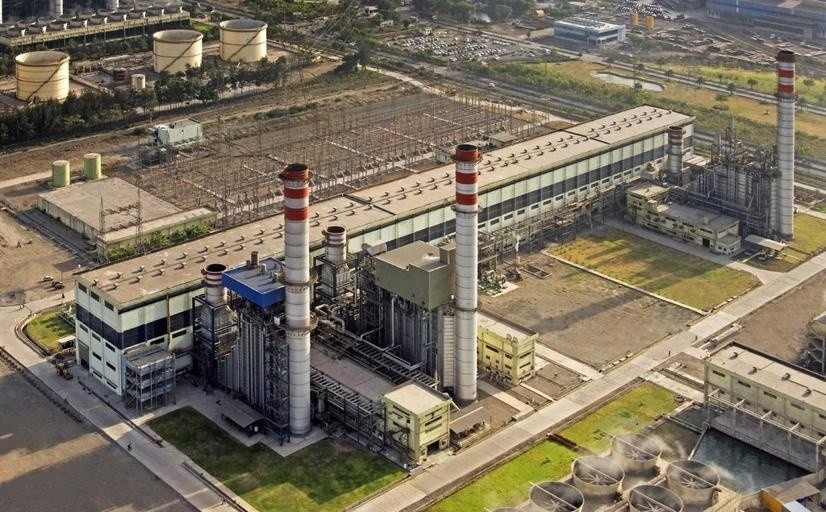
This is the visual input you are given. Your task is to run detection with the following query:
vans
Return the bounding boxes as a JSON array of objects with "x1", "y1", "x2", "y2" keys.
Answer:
[
  {"x1": 52, "y1": 281, "x2": 63, "y2": 287},
  {"x1": 54, "y1": 283, "x2": 65, "y2": 290},
  {"x1": 43, "y1": 276, "x2": 54, "y2": 282}
]
